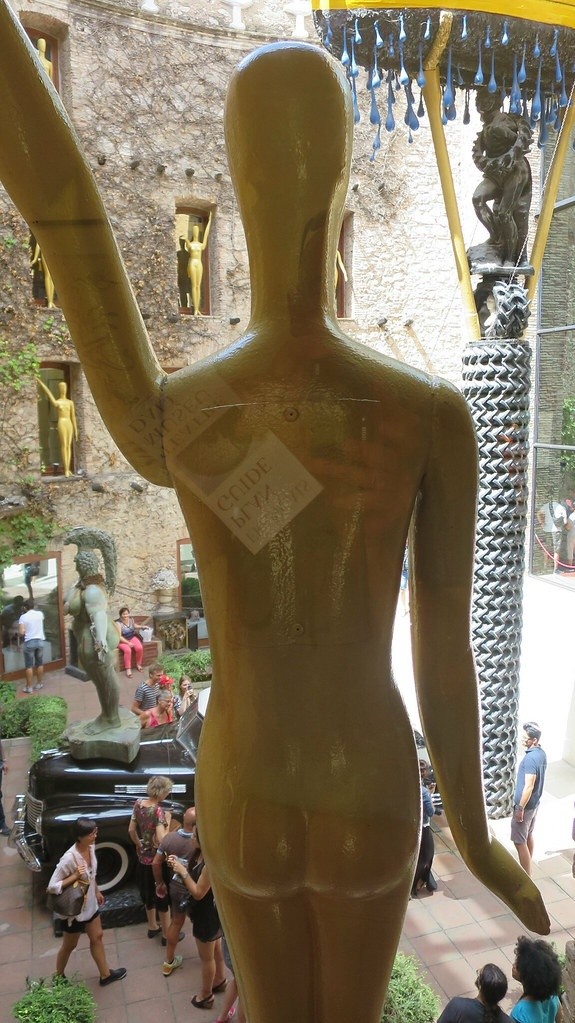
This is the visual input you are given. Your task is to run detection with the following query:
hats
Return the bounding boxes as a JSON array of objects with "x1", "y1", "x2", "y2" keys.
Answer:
[{"x1": 158, "y1": 675, "x2": 174, "y2": 685}]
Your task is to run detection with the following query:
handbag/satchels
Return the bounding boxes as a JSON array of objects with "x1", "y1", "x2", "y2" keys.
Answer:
[
  {"x1": 45, "y1": 851, "x2": 92, "y2": 917},
  {"x1": 553, "y1": 516, "x2": 564, "y2": 528},
  {"x1": 133, "y1": 631, "x2": 143, "y2": 642}
]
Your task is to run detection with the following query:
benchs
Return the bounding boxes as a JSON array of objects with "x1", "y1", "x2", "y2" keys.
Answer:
[{"x1": 112, "y1": 641, "x2": 163, "y2": 673}]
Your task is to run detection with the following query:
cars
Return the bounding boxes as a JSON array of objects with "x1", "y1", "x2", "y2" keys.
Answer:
[{"x1": 4, "y1": 685, "x2": 445, "y2": 898}]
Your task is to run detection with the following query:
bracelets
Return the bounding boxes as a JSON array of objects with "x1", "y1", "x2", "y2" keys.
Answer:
[
  {"x1": 3, "y1": 759, "x2": 5, "y2": 762},
  {"x1": 155, "y1": 882, "x2": 163, "y2": 887},
  {"x1": 181, "y1": 872, "x2": 189, "y2": 879}
]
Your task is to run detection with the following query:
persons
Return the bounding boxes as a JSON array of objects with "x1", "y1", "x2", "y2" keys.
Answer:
[
  {"x1": 411, "y1": 759, "x2": 438, "y2": 897},
  {"x1": 1, "y1": 596, "x2": 24, "y2": 649},
  {"x1": 538, "y1": 489, "x2": 575, "y2": 574},
  {"x1": 47, "y1": 816, "x2": 126, "y2": 989},
  {"x1": 400, "y1": 539, "x2": 409, "y2": 589},
  {"x1": 335, "y1": 251, "x2": 347, "y2": 288},
  {"x1": 19, "y1": 563, "x2": 33, "y2": 600},
  {"x1": 129, "y1": 775, "x2": 238, "y2": 1023},
  {"x1": 62, "y1": 526, "x2": 121, "y2": 735},
  {"x1": 131, "y1": 664, "x2": 195, "y2": 729},
  {"x1": 16, "y1": 599, "x2": 44, "y2": 693},
  {"x1": 436, "y1": 935, "x2": 562, "y2": 1023},
  {"x1": 510, "y1": 721, "x2": 547, "y2": 876},
  {"x1": 37, "y1": 377, "x2": 78, "y2": 478},
  {"x1": 0, "y1": 0, "x2": 547, "y2": 1023},
  {"x1": 180, "y1": 210, "x2": 211, "y2": 315},
  {"x1": 471, "y1": 90, "x2": 535, "y2": 258},
  {"x1": 115, "y1": 608, "x2": 149, "y2": 677},
  {"x1": 0, "y1": 703, "x2": 12, "y2": 835},
  {"x1": 31, "y1": 242, "x2": 56, "y2": 309}
]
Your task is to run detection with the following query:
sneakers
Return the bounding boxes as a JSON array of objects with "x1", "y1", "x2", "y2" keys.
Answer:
[
  {"x1": 100, "y1": 967, "x2": 127, "y2": 986},
  {"x1": 161, "y1": 954, "x2": 183, "y2": 976},
  {"x1": 55, "y1": 974, "x2": 68, "y2": 983},
  {"x1": 214, "y1": 1007, "x2": 235, "y2": 1023}
]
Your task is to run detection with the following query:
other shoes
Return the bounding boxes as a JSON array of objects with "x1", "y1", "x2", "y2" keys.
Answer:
[
  {"x1": 544, "y1": 565, "x2": 548, "y2": 569},
  {"x1": 126, "y1": 670, "x2": 133, "y2": 678},
  {"x1": 33, "y1": 683, "x2": 44, "y2": 690},
  {"x1": 136, "y1": 665, "x2": 144, "y2": 672},
  {"x1": 553, "y1": 569, "x2": 564, "y2": 574},
  {"x1": 148, "y1": 924, "x2": 162, "y2": 938},
  {"x1": 23, "y1": 687, "x2": 33, "y2": 692},
  {"x1": 0, "y1": 825, "x2": 13, "y2": 835},
  {"x1": 162, "y1": 932, "x2": 185, "y2": 946}
]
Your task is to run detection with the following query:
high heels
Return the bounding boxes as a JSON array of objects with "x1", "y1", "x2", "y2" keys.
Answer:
[
  {"x1": 191, "y1": 993, "x2": 215, "y2": 1009},
  {"x1": 212, "y1": 978, "x2": 228, "y2": 992}
]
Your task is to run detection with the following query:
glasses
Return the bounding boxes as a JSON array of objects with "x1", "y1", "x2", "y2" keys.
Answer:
[{"x1": 522, "y1": 735, "x2": 536, "y2": 740}]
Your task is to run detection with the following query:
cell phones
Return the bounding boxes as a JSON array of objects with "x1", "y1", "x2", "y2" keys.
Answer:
[
  {"x1": 188, "y1": 685, "x2": 192, "y2": 690},
  {"x1": 179, "y1": 858, "x2": 187, "y2": 867}
]
